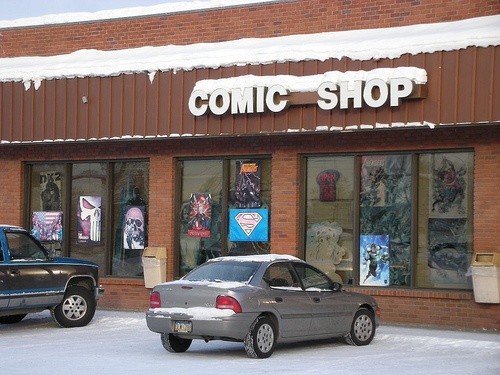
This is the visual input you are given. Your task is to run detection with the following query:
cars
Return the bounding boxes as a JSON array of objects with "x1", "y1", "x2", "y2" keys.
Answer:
[
  {"x1": 147, "y1": 253, "x2": 382, "y2": 355},
  {"x1": 1, "y1": 224, "x2": 104, "y2": 329}
]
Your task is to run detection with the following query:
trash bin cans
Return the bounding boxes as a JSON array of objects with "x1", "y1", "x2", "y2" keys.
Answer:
[
  {"x1": 470, "y1": 250, "x2": 500, "y2": 303},
  {"x1": 141, "y1": 246, "x2": 167, "y2": 289}
]
